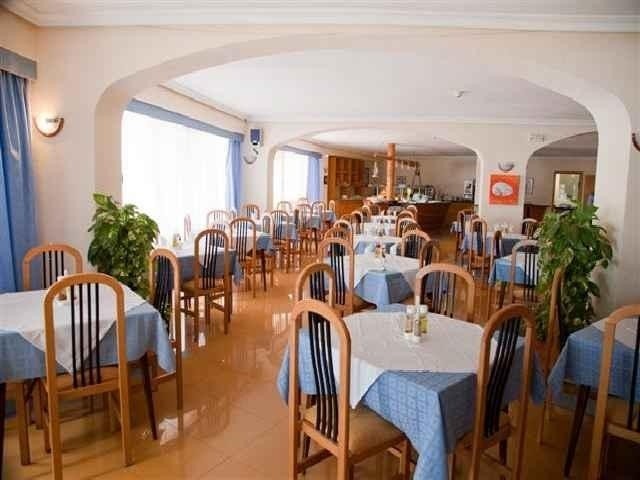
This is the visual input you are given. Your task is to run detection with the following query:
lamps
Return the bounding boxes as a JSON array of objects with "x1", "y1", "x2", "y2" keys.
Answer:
[
  {"x1": 32, "y1": 111, "x2": 65, "y2": 136},
  {"x1": 244, "y1": 155, "x2": 258, "y2": 165},
  {"x1": 498, "y1": 160, "x2": 515, "y2": 173}
]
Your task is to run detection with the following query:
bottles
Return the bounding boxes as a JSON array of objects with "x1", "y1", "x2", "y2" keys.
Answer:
[
  {"x1": 170, "y1": 231, "x2": 181, "y2": 248},
  {"x1": 57, "y1": 268, "x2": 78, "y2": 303},
  {"x1": 374, "y1": 245, "x2": 387, "y2": 260},
  {"x1": 404, "y1": 304, "x2": 430, "y2": 343}
]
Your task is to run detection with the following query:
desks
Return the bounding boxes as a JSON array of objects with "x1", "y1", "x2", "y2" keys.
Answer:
[
  {"x1": 296, "y1": 306, "x2": 524, "y2": 479},
  {"x1": 1, "y1": 281, "x2": 160, "y2": 444}
]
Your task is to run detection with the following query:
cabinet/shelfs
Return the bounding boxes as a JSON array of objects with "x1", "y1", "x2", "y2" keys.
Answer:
[{"x1": 328, "y1": 152, "x2": 368, "y2": 205}]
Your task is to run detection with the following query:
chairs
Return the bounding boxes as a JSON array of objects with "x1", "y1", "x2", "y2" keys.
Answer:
[
  {"x1": 293, "y1": 264, "x2": 338, "y2": 325},
  {"x1": 444, "y1": 302, "x2": 531, "y2": 480},
  {"x1": 4, "y1": 378, "x2": 32, "y2": 466},
  {"x1": 37, "y1": 272, "x2": 133, "y2": 479},
  {"x1": 19, "y1": 243, "x2": 83, "y2": 431},
  {"x1": 534, "y1": 264, "x2": 639, "y2": 480},
  {"x1": 98, "y1": 246, "x2": 181, "y2": 431},
  {"x1": 141, "y1": 196, "x2": 548, "y2": 342},
  {"x1": 288, "y1": 300, "x2": 409, "y2": 479}
]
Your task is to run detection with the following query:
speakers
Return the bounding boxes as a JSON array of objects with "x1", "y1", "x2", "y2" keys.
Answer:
[{"x1": 248, "y1": 128, "x2": 262, "y2": 148}]
